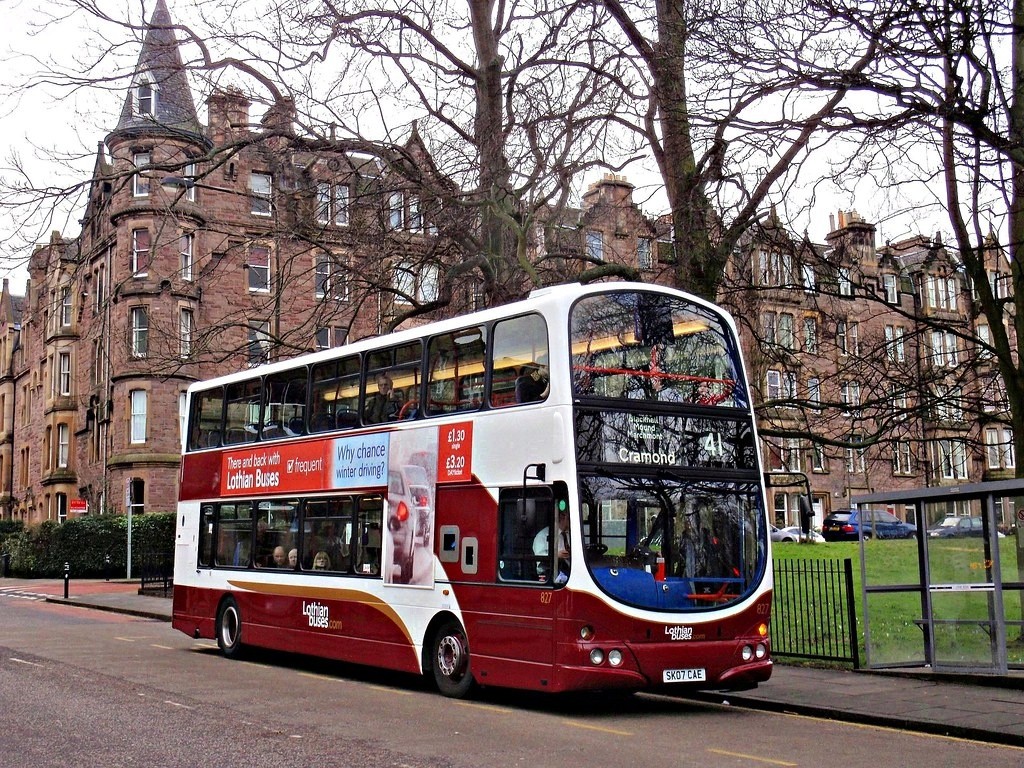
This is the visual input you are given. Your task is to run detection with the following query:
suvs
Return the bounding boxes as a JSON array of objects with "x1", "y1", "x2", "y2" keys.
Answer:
[{"x1": 821, "y1": 507, "x2": 917, "y2": 542}]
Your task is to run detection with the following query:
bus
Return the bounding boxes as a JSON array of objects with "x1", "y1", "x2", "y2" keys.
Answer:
[{"x1": 171, "y1": 281, "x2": 816, "y2": 706}]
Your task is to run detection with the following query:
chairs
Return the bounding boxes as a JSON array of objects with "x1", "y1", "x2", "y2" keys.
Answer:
[{"x1": 190, "y1": 375, "x2": 742, "y2": 608}]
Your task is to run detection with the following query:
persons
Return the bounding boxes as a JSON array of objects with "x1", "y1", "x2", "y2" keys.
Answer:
[
  {"x1": 324, "y1": 525, "x2": 344, "y2": 547},
  {"x1": 312, "y1": 553, "x2": 331, "y2": 571},
  {"x1": 515, "y1": 355, "x2": 550, "y2": 404},
  {"x1": 687, "y1": 381, "x2": 732, "y2": 405},
  {"x1": 272, "y1": 545, "x2": 287, "y2": 569},
  {"x1": 532, "y1": 504, "x2": 570, "y2": 582},
  {"x1": 287, "y1": 549, "x2": 298, "y2": 569},
  {"x1": 367, "y1": 376, "x2": 404, "y2": 423}
]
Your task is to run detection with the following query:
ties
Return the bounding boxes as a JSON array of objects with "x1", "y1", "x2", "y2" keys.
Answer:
[{"x1": 561, "y1": 532, "x2": 571, "y2": 553}]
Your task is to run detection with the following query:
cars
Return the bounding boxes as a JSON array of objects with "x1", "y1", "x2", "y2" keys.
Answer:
[
  {"x1": 770, "y1": 524, "x2": 797, "y2": 542},
  {"x1": 781, "y1": 526, "x2": 826, "y2": 542},
  {"x1": 926, "y1": 515, "x2": 983, "y2": 539}
]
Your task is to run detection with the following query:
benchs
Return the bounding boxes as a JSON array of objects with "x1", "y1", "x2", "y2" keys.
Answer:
[{"x1": 912, "y1": 619, "x2": 1024, "y2": 675}]
